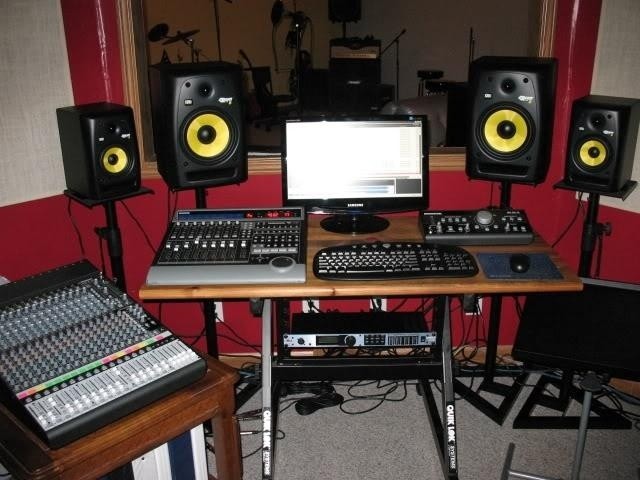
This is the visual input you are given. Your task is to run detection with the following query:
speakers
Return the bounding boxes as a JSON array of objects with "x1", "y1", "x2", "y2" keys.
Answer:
[
  {"x1": 148, "y1": 62, "x2": 248, "y2": 189},
  {"x1": 564, "y1": 95, "x2": 640, "y2": 191},
  {"x1": 330, "y1": 40, "x2": 381, "y2": 113},
  {"x1": 56, "y1": 102, "x2": 142, "y2": 200},
  {"x1": 461, "y1": 56, "x2": 559, "y2": 186}
]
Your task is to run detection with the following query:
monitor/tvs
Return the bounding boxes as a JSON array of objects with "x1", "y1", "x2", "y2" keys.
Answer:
[{"x1": 280, "y1": 114, "x2": 429, "y2": 235}]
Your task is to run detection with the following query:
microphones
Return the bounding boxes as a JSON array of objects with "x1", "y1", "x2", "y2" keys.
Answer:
[{"x1": 396, "y1": 26, "x2": 406, "y2": 39}]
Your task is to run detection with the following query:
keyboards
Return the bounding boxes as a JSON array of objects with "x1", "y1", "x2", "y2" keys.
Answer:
[{"x1": 313, "y1": 242, "x2": 479, "y2": 278}]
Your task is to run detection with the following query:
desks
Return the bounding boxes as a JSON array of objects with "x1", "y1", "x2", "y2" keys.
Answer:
[
  {"x1": 138, "y1": 209, "x2": 586, "y2": 480},
  {"x1": 2, "y1": 347, "x2": 245, "y2": 480}
]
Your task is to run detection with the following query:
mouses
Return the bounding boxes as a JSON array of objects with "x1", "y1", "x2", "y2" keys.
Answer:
[{"x1": 510, "y1": 253, "x2": 531, "y2": 273}]
